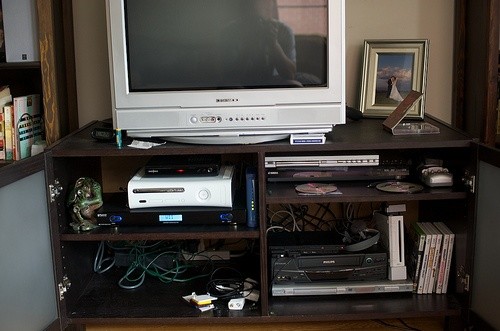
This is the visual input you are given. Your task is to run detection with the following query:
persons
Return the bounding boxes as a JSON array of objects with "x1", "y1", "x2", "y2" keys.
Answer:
[
  {"x1": 386, "y1": 76, "x2": 405, "y2": 101},
  {"x1": 228, "y1": 0, "x2": 302, "y2": 88}
]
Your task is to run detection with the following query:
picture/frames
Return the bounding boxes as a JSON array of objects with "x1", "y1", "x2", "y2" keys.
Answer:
[{"x1": 359, "y1": 39, "x2": 430, "y2": 120}]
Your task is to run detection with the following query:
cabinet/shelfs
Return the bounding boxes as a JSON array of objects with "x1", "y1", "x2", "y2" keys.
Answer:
[{"x1": 43, "y1": 112, "x2": 482, "y2": 331}]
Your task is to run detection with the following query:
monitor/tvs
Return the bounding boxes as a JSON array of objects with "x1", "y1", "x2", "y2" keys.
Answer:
[{"x1": 104, "y1": 0, "x2": 346, "y2": 145}]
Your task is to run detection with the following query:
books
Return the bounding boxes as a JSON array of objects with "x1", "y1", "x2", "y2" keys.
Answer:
[
  {"x1": 0, "y1": 86, "x2": 43, "y2": 161},
  {"x1": 411, "y1": 219, "x2": 455, "y2": 295}
]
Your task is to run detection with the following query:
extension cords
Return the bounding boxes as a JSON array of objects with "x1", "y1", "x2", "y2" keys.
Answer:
[{"x1": 179, "y1": 249, "x2": 231, "y2": 261}]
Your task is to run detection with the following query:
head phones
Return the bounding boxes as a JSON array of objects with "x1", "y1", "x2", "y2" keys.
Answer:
[{"x1": 333, "y1": 220, "x2": 381, "y2": 252}]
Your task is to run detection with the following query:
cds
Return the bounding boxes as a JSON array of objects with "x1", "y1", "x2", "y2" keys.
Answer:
[
  {"x1": 296, "y1": 183, "x2": 338, "y2": 193},
  {"x1": 376, "y1": 181, "x2": 422, "y2": 193}
]
